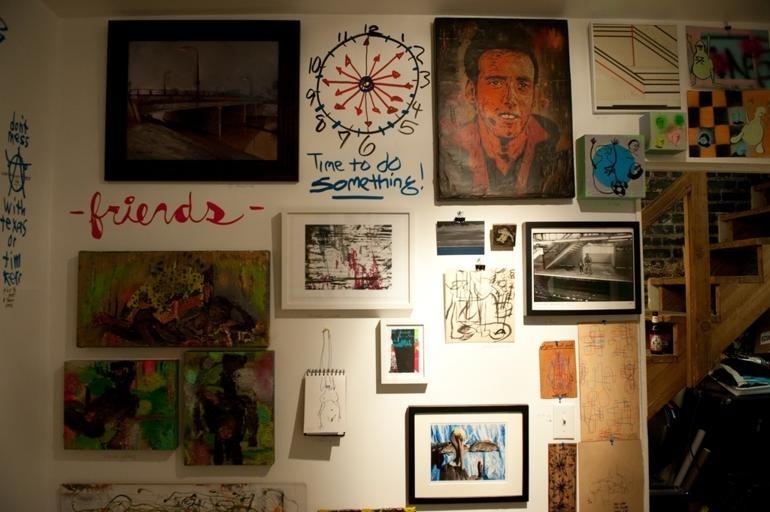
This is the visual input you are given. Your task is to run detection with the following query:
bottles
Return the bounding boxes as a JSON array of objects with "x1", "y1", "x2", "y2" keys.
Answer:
[{"x1": 648, "y1": 310, "x2": 664, "y2": 355}]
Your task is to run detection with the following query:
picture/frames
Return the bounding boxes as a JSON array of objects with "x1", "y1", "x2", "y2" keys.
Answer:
[
  {"x1": 103, "y1": 16, "x2": 302, "y2": 188},
  {"x1": 406, "y1": 404, "x2": 530, "y2": 506},
  {"x1": 378, "y1": 319, "x2": 429, "y2": 387},
  {"x1": 521, "y1": 221, "x2": 644, "y2": 318},
  {"x1": 278, "y1": 205, "x2": 416, "y2": 309}
]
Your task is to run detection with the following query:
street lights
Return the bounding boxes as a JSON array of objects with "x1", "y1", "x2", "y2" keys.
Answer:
[
  {"x1": 163, "y1": 70, "x2": 171, "y2": 95},
  {"x1": 243, "y1": 77, "x2": 253, "y2": 96},
  {"x1": 176, "y1": 45, "x2": 199, "y2": 96}
]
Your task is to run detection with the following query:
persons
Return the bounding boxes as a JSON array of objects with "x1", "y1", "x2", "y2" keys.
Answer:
[
  {"x1": 578, "y1": 258, "x2": 584, "y2": 273},
  {"x1": 584, "y1": 252, "x2": 593, "y2": 276},
  {"x1": 448, "y1": 22, "x2": 562, "y2": 197},
  {"x1": 317, "y1": 377, "x2": 341, "y2": 431}
]
